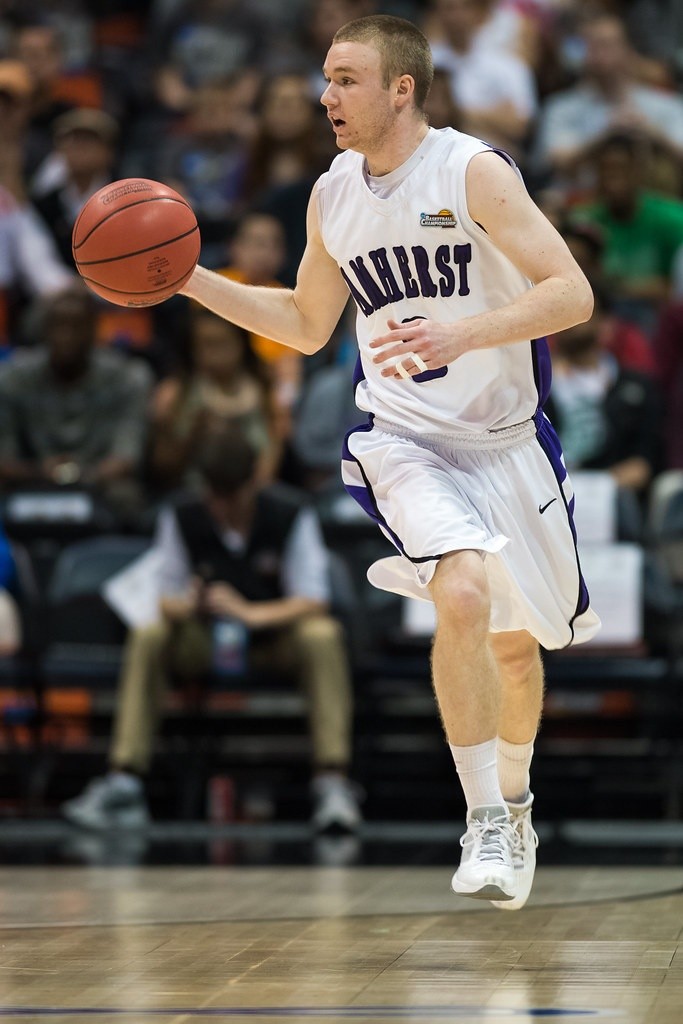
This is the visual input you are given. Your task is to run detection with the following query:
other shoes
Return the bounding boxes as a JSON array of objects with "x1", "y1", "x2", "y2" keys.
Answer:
[
  {"x1": 62, "y1": 767, "x2": 149, "y2": 828},
  {"x1": 312, "y1": 775, "x2": 362, "y2": 836}
]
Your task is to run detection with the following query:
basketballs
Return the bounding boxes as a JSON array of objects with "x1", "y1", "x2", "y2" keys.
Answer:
[{"x1": 72, "y1": 179, "x2": 202, "y2": 309}]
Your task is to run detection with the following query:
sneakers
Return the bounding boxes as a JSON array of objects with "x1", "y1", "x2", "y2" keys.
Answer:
[
  {"x1": 452, "y1": 804, "x2": 520, "y2": 900},
  {"x1": 491, "y1": 791, "x2": 539, "y2": 911}
]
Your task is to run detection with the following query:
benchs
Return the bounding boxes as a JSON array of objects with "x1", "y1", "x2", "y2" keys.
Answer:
[{"x1": 3, "y1": 686, "x2": 630, "y2": 866}]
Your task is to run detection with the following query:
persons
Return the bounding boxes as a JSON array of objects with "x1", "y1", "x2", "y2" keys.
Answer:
[
  {"x1": 174, "y1": 15, "x2": 596, "y2": 911},
  {"x1": 0, "y1": 0, "x2": 682, "y2": 837}
]
